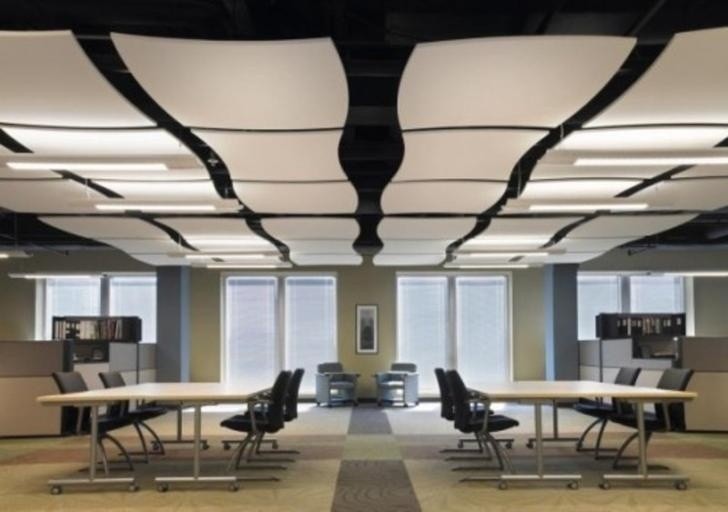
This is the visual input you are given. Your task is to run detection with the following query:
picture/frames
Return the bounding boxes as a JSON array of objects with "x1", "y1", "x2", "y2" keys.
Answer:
[{"x1": 353, "y1": 303, "x2": 379, "y2": 356}]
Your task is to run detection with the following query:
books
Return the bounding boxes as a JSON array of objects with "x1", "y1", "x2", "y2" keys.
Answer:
[
  {"x1": 615, "y1": 315, "x2": 683, "y2": 338},
  {"x1": 54, "y1": 319, "x2": 135, "y2": 341}
]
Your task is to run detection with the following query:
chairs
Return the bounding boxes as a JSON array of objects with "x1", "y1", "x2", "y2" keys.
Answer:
[
  {"x1": 37, "y1": 359, "x2": 307, "y2": 497},
  {"x1": 430, "y1": 364, "x2": 698, "y2": 494},
  {"x1": 373, "y1": 361, "x2": 422, "y2": 409},
  {"x1": 314, "y1": 360, "x2": 362, "y2": 409}
]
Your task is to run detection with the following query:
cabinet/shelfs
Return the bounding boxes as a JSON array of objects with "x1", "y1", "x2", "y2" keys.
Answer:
[
  {"x1": 594, "y1": 310, "x2": 686, "y2": 357},
  {"x1": 50, "y1": 315, "x2": 142, "y2": 363}
]
